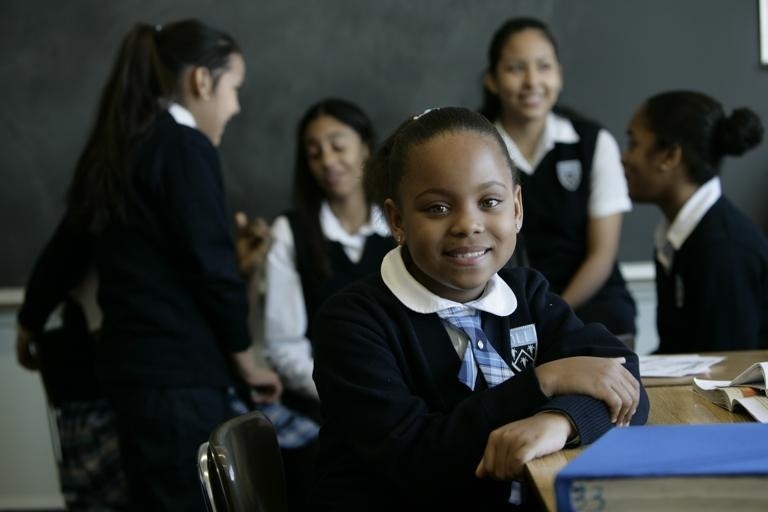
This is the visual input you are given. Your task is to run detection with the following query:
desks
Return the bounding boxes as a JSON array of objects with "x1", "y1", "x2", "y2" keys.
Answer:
[{"x1": 523, "y1": 349, "x2": 767, "y2": 512}]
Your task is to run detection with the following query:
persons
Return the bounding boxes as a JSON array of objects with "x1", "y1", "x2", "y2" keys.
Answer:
[
  {"x1": 473, "y1": 14, "x2": 638, "y2": 352},
  {"x1": 308, "y1": 104, "x2": 652, "y2": 511},
  {"x1": 261, "y1": 95, "x2": 404, "y2": 419},
  {"x1": 60, "y1": 210, "x2": 280, "y2": 344},
  {"x1": 620, "y1": 86, "x2": 768, "y2": 353},
  {"x1": 12, "y1": 15, "x2": 286, "y2": 511}
]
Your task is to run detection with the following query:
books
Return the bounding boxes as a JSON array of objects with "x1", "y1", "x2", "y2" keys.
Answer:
[{"x1": 553, "y1": 350, "x2": 768, "y2": 512}]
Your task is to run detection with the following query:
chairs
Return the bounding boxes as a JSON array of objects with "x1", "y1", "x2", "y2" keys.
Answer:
[{"x1": 195, "y1": 411, "x2": 286, "y2": 512}]
